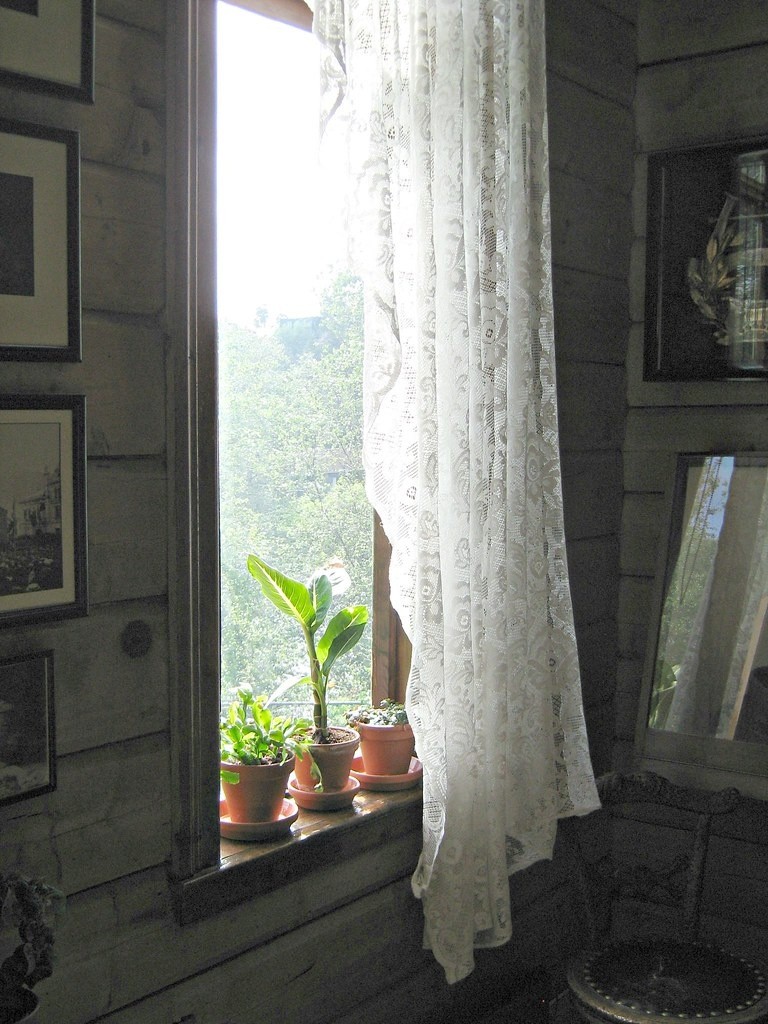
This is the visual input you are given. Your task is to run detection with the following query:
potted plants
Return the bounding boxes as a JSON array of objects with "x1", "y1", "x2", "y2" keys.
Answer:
[{"x1": 218, "y1": 551, "x2": 425, "y2": 843}]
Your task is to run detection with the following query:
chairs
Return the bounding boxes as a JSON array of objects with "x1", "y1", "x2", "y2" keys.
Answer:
[{"x1": 567, "y1": 766, "x2": 768, "y2": 1024}]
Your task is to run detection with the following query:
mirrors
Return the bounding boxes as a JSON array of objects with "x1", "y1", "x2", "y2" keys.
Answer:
[{"x1": 632, "y1": 450, "x2": 768, "y2": 800}]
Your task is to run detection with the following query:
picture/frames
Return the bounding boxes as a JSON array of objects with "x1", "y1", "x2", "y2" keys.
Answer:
[
  {"x1": 623, "y1": 123, "x2": 768, "y2": 407},
  {"x1": 0, "y1": 0, "x2": 96, "y2": 107},
  {"x1": 0, "y1": 647, "x2": 58, "y2": 808},
  {"x1": 0, "y1": 393, "x2": 90, "y2": 629},
  {"x1": 0, "y1": 118, "x2": 82, "y2": 363}
]
